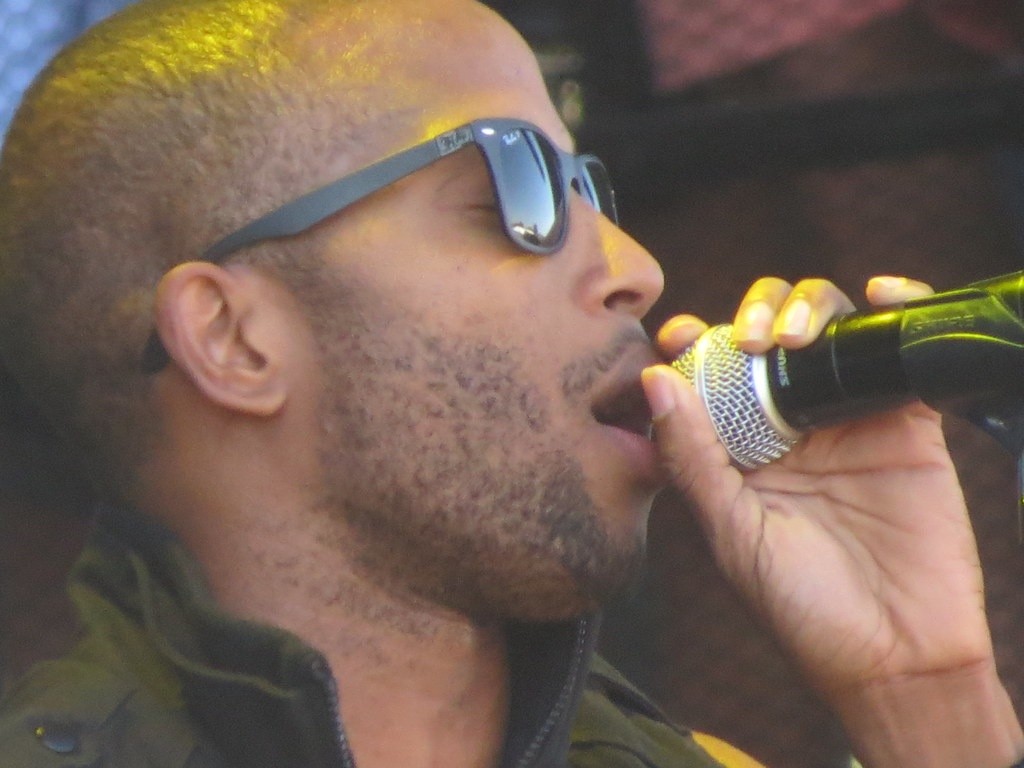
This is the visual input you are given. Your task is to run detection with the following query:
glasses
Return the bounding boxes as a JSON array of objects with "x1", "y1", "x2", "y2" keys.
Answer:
[{"x1": 133, "y1": 117, "x2": 620, "y2": 373}]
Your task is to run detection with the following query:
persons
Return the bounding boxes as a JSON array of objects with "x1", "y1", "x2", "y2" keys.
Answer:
[{"x1": 0, "y1": 0, "x2": 1024, "y2": 768}]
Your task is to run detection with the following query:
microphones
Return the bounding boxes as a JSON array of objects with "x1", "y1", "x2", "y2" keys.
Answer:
[{"x1": 647, "y1": 271, "x2": 1024, "y2": 475}]
